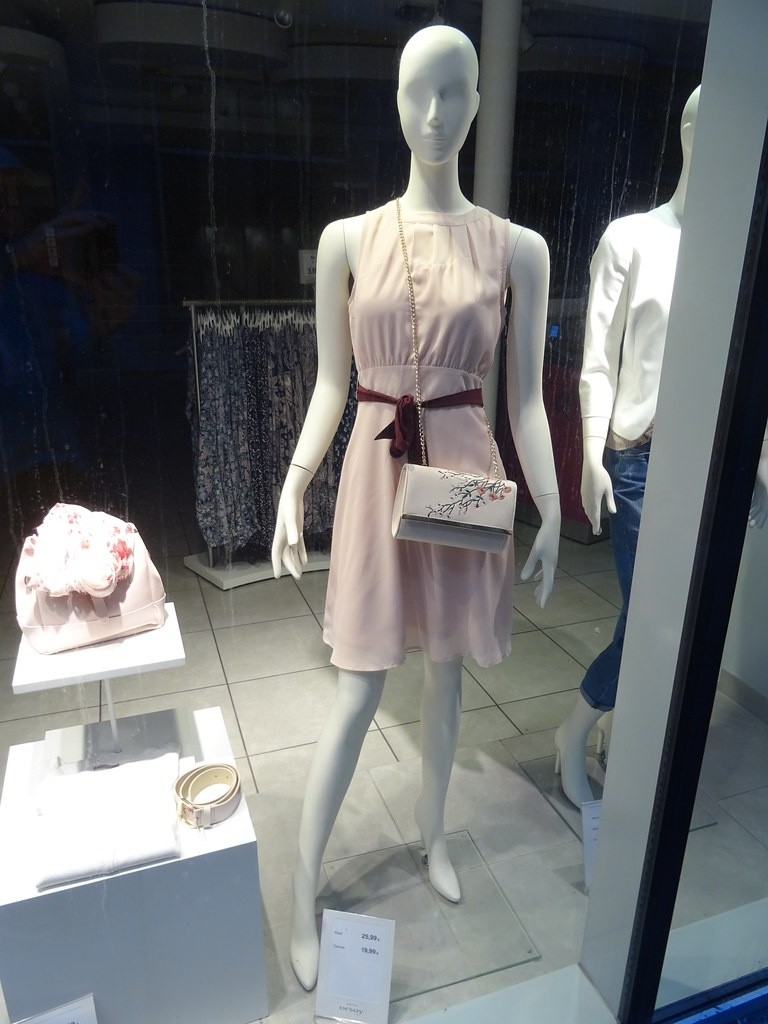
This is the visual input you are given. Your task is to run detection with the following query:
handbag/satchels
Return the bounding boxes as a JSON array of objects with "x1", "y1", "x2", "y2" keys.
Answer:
[
  {"x1": 14, "y1": 524, "x2": 165, "y2": 656},
  {"x1": 392, "y1": 463, "x2": 517, "y2": 556}
]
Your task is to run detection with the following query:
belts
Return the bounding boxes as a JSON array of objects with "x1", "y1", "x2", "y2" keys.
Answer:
[{"x1": 174, "y1": 762, "x2": 243, "y2": 830}]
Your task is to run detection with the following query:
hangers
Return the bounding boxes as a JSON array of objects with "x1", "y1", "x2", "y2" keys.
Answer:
[{"x1": 195, "y1": 298, "x2": 316, "y2": 344}]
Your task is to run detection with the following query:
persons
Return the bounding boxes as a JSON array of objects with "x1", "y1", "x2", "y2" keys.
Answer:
[
  {"x1": 553, "y1": 82, "x2": 768, "y2": 812},
  {"x1": 272, "y1": 23, "x2": 563, "y2": 991}
]
